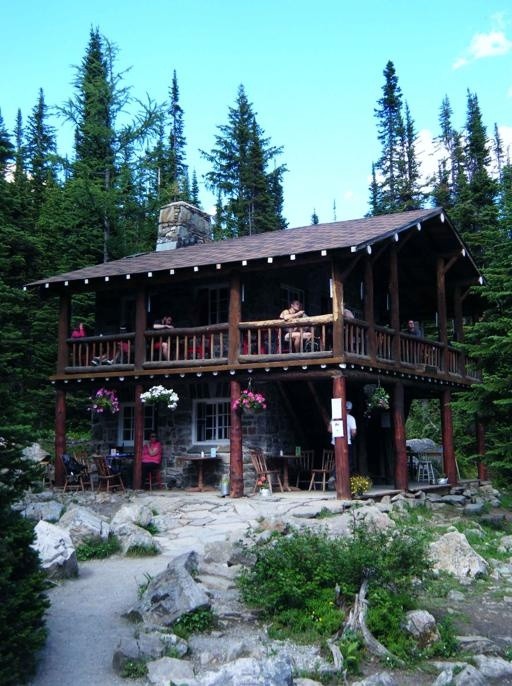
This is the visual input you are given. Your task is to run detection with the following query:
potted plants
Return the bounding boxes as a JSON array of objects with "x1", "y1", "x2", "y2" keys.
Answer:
[{"x1": 362, "y1": 385, "x2": 389, "y2": 418}]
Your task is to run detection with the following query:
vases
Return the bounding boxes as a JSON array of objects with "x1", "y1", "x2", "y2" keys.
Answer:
[
  {"x1": 358, "y1": 491, "x2": 363, "y2": 495},
  {"x1": 243, "y1": 405, "x2": 256, "y2": 414}
]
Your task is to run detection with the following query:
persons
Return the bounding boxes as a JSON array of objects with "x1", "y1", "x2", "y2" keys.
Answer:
[
  {"x1": 90, "y1": 326, "x2": 128, "y2": 366},
  {"x1": 403, "y1": 319, "x2": 422, "y2": 363},
  {"x1": 274, "y1": 300, "x2": 312, "y2": 353},
  {"x1": 326, "y1": 400, "x2": 358, "y2": 491},
  {"x1": 342, "y1": 308, "x2": 355, "y2": 348},
  {"x1": 151, "y1": 315, "x2": 174, "y2": 361},
  {"x1": 71, "y1": 320, "x2": 90, "y2": 353},
  {"x1": 126, "y1": 431, "x2": 163, "y2": 490}
]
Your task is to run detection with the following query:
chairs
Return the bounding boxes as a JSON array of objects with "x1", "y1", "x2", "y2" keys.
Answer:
[
  {"x1": 248, "y1": 443, "x2": 335, "y2": 492},
  {"x1": 415, "y1": 445, "x2": 436, "y2": 483},
  {"x1": 36, "y1": 451, "x2": 162, "y2": 497},
  {"x1": 178, "y1": 327, "x2": 293, "y2": 356}
]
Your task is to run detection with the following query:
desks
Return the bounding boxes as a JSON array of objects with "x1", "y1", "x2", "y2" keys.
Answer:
[{"x1": 407, "y1": 451, "x2": 443, "y2": 479}]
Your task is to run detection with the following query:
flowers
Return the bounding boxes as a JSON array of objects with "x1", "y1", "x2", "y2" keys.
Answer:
[
  {"x1": 231, "y1": 389, "x2": 267, "y2": 412},
  {"x1": 139, "y1": 384, "x2": 179, "y2": 411},
  {"x1": 84, "y1": 386, "x2": 119, "y2": 414},
  {"x1": 349, "y1": 473, "x2": 371, "y2": 494}
]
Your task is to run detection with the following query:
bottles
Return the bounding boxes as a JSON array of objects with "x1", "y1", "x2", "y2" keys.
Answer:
[
  {"x1": 116, "y1": 450, "x2": 120, "y2": 455},
  {"x1": 280, "y1": 448, "x2": 284, "y2": 456},
  {"x1": 200, "y1": 450, "x2": 205, "y2": 458}
]
